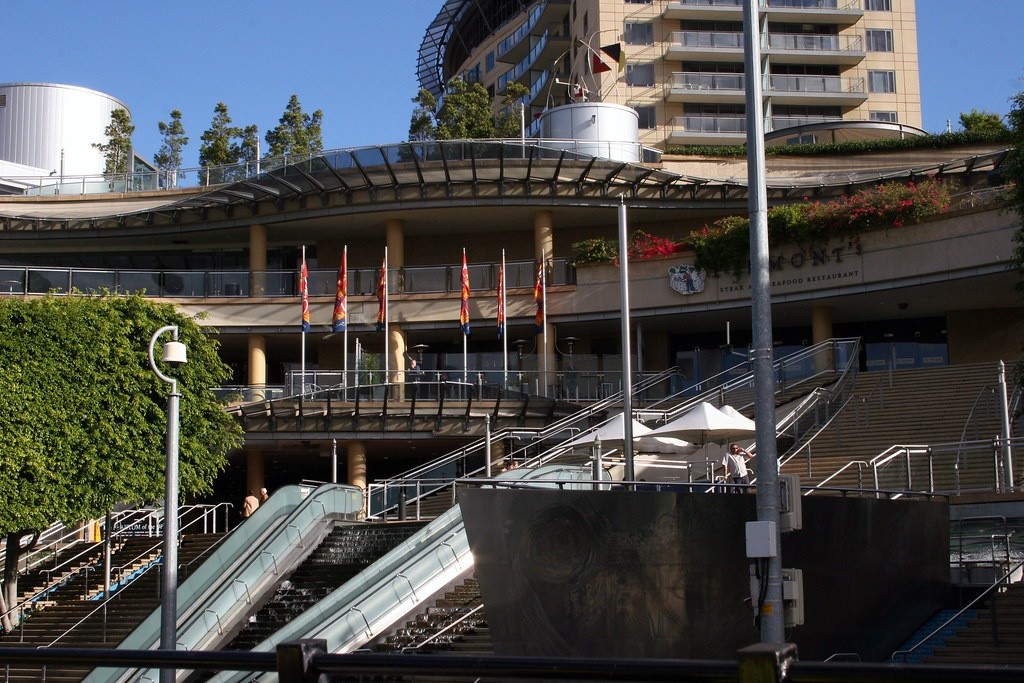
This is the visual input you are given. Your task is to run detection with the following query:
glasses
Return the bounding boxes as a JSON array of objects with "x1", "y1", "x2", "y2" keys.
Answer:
[{"x1": 731, "y1": 447, "x2": 738, "y2": 449}]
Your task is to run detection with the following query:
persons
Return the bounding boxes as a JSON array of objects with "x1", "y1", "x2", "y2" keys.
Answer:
[
  {"x1": 407, "y1": 359, "x2": 421, "y2": 398},
  {"x1": 722, "y1": 444, "x2": 754, "y2": 493},
  {"x1": 502, "y1": 461, "x2": 519, "y2": 473},
  {"x1": 243, "y1": 488, "x2": 268, "y2": 520}
]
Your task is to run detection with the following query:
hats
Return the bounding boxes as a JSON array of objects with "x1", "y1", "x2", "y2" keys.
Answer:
[{"x1": 261, "y1": 487, "x2": 267, "y2": 493}]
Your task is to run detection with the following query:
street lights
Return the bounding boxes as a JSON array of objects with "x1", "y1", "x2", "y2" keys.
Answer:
[{"x1": 149, "y1": 325, "x2": 188, "y2": 683}]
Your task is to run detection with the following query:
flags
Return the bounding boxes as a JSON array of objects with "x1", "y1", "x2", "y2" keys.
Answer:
[
  {"x1": 497, "y1": 259, "x2": 503, "y2": 339},
  {"x1": 332, "y1": 249, "x2": 345, "y2": 332},
  {"x1": 300, "y1": 249, "x2": 310, "y2": 335},
  {"x1": 534, "y1": 259, "x2": 545, "y2": 334},
  {"x1": 374, "y1": 258, "x2": 387, "y2": 331},
  {"x1": 460, "y1": 253, "x2": 471, "y2": 334}
]
